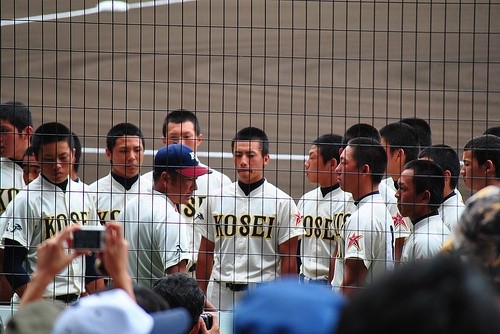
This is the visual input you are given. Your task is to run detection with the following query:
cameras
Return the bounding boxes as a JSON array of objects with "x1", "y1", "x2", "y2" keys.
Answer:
[
  {"x1": 198, "y1": 314, "x2": 212, "y2": 334},
  {"x1": 72, "y1": 225, "x2": 108, "y2": 252}
]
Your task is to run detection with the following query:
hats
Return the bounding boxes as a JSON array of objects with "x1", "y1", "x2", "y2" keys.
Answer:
[
  {"x1": 54, "y1": 288, "x2": 190, "y2": 334},
  {"x1": 236, "y1": 280, "x2": 346, "y2": 334},
  {"x1": 154, "y1": 141, "x2": 208, "y2": 178},
  {"x1": 6, "y1": 299, "x2": 66, "y2": 334},
  {"x1": 462, "y1": 185, "x2": 500, "y2": 245}
]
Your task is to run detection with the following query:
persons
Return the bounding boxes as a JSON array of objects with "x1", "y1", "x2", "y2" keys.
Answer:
[
  {"x1": 111, "y1": 142, "x2": 209, "y2": 289},
  {"x1": 0, "y1": 100, "x2": 500, "y2": 334},
  {"x1": 195, "y1": 127, "x2": 306, "y2": 311}
]
